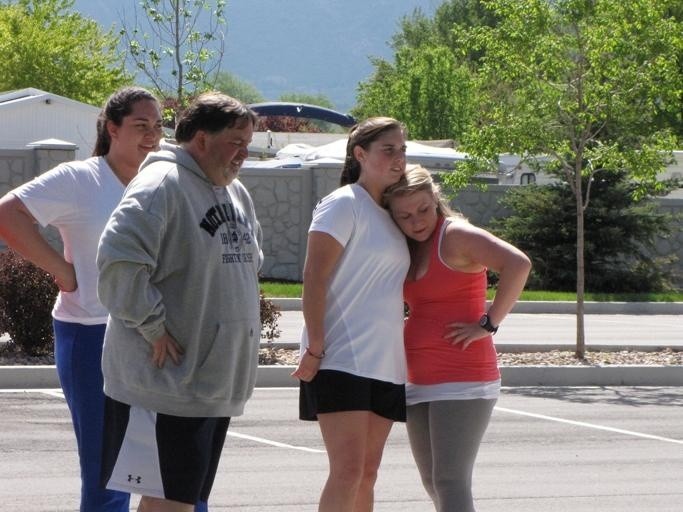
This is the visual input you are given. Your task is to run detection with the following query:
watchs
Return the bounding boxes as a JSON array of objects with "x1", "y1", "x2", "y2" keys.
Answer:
[{"x1": 480, "y1": 312, "x2": 499, "y2": 336}]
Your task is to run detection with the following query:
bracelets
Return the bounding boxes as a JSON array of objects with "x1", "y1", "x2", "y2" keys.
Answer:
[{"x1": 306, "y1": 346, "x2": 327, "y2": 360}]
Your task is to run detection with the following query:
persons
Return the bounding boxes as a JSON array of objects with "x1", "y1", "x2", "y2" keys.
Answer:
[
  {"x1": 289, "y1": 117, "x2": 413, "y2": 512},
  {"x1": 95, "y1": 92, "x2": 263, "y2": 512},
  {"x1": 0, "y1": 89, "x2": 209, "y2": 510},
  {"x1": 383, "y1": 164, "x2": 533, "y2": 512}
]
